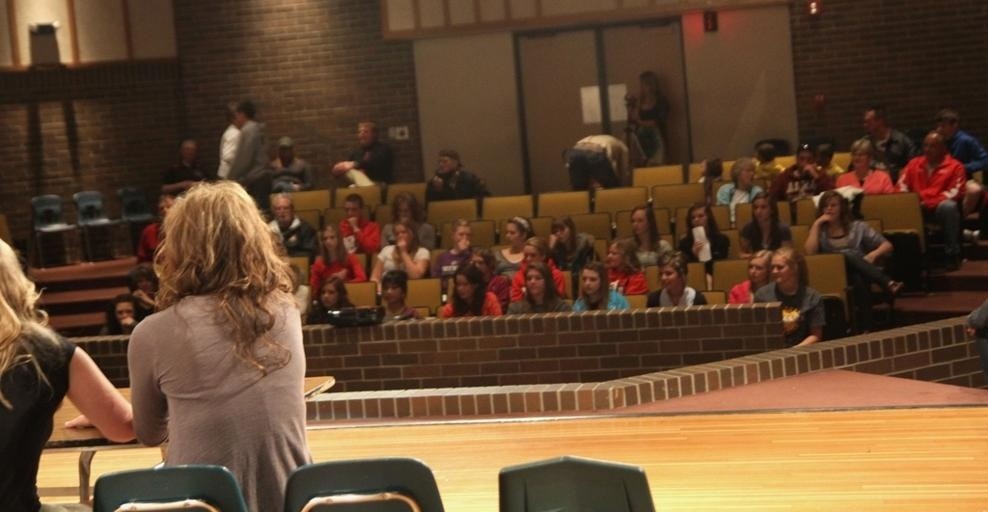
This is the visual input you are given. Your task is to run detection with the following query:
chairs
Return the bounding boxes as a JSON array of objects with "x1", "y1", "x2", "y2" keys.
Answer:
[
  {"x1": 117, "y1": 186, "x2": 155, "y2": 255},
  {"x1": 282, "y1": 449, "x2": 445, "y2": 511},
  {"x1": 89, "y1": 463, "x2": 251, "y2": 510},
  {"x1": 498, "y1": 454, "x2": 653, "y2": 512},
  {"x1": 73, "y1": 189, "x2": 114, "y2": 260},
  {"x1": 155, "y1": 153, "x2": 983, "y2": 316},
  {"x1": 31, "y1": 194, "x2": 76, "y2": 265}
]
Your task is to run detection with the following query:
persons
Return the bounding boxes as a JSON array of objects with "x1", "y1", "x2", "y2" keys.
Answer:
[
  {"x1": 162, "y1": 140, "x2": 209, "y2": 199},
  {"x1": 217, "y1": 100, "x2": 315, "y2": 220},
  {"x1": 332, "y1": 118, "x2": 393, "y2": 205},
  {"x1": 0, "y1": 234, "x2": 139, "y2": 512},
  {"x1": 565, "y1": 72, "x2": 671, "y2": 212},
  {"x1": 105, "y1": 178, "x2": 601, "y2": 328},
  {"x1": 425, "y1": 149, "x2": 494, "y2": 219},
  {"x1": 570, "y1": 189, "x2": 827, "y2": 348},
  {"x1": 127, "y1": 180, "x2": 312, "y2": 511},
  {"x1": 697, "y1": 105, "x2": 987, "y2": 334}
]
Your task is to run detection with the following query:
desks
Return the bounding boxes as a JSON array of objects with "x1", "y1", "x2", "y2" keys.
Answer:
[{"x1": 42, "y1": 376, "x2": 337, "y2": 502}]
